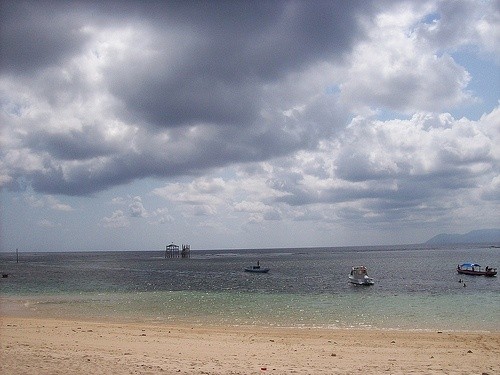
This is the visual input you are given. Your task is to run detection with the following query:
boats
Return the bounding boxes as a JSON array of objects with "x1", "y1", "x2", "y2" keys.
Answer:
[
  {"x1": 457, "y1": 263, "x2": 498, "y2": 276},
  {"x1": 347, "y1": 266, "x2": 373, "y2": 285},
  {"x1": 244, "y1": 265, "x2": 271, "y2": 273}
]
